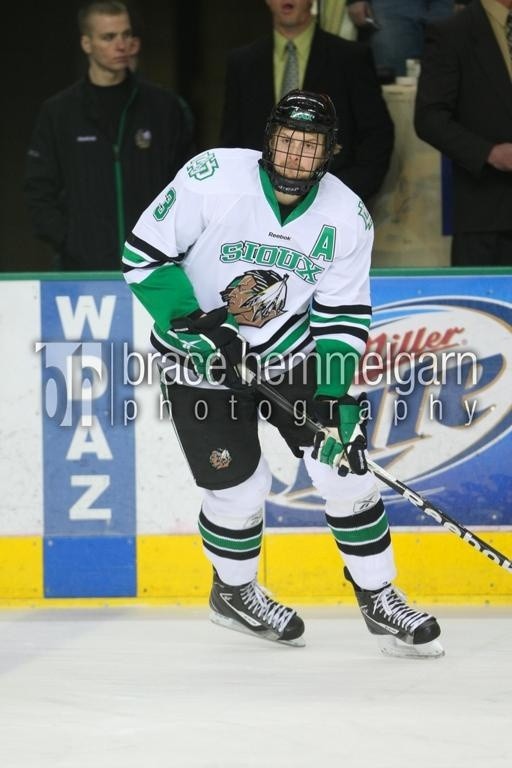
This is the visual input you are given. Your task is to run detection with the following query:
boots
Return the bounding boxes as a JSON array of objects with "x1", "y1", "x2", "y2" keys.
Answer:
[
  {"x1": 207, "y1": 562, "x2": 306, "y2": 642},
  {"x1": 341, "y1": 564, "x2": 440, "y2": 646}
]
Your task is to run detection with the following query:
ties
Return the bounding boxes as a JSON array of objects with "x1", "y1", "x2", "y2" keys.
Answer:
[
  {"x1": 277, "y1": 42, "x2": 300, "y2": 101},
  {"x1": 505, "y1": 13, "x2": 512, "y2": 61}
]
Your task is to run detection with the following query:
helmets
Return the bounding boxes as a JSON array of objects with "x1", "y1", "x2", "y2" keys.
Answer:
[{"x1": 257, "y1": 88, "x2": 339, "y2": 197}]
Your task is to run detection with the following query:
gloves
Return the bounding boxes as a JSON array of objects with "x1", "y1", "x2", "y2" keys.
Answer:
[
  {"x1": 309, "y1": 390, "x2": 372, "y2": 479},
  {"x1": 170, "y1": 304, "x2": 261, "y2": 397}
]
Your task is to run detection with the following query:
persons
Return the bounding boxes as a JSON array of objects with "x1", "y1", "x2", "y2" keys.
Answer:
[
  {"x1": 413, "y1": 0, "x2": 512, "y2": 266},
  {"x1": 118, "y1": 89, "x2": 441, "y2": 644},
  {"x1": 220, "y1": 1, "x2": 394, "y2": 203},
  {"x1": 345, "y1": 0, "x2": 457, "y2": 81},
  {"x1": 13, "y1": 1, "x2": 194, "y2": 274}
]
